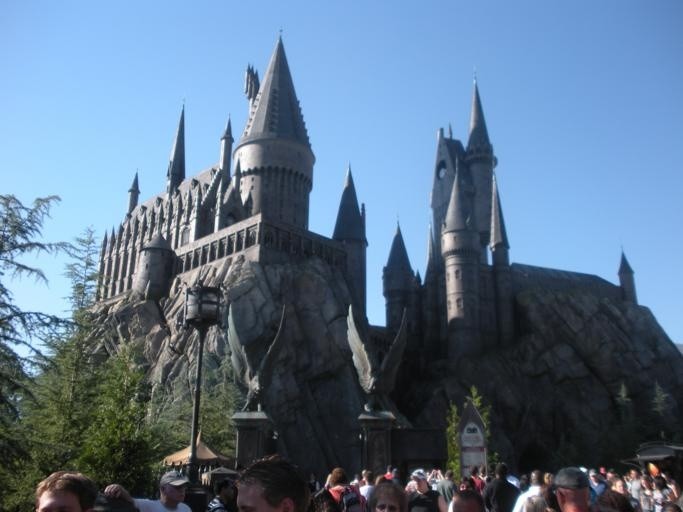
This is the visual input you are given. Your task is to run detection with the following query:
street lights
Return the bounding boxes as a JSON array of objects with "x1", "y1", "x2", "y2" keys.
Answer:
[{"x1": 185, "y1": 280, "x2": 222, "y2": 487}]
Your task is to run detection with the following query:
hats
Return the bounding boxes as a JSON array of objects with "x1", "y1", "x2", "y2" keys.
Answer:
[
  {"x1": 160, "y1": 470, "x2": 191, "y2": 488},
  {"x1": 555, "y1": 467, "x2": 590, "y2": 490},
  {"x1": 410, "y1": 468, "x2": 427, "y2": 480}
]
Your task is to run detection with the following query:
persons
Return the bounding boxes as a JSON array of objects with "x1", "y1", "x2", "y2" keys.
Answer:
[{"x1": 35, "y1": 455, "x2": 683, "y2": 511}]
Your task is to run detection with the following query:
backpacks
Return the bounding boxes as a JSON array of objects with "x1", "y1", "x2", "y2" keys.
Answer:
[{"x1": 341, "y1": 486, "x2": 360, "y2": 512}]
